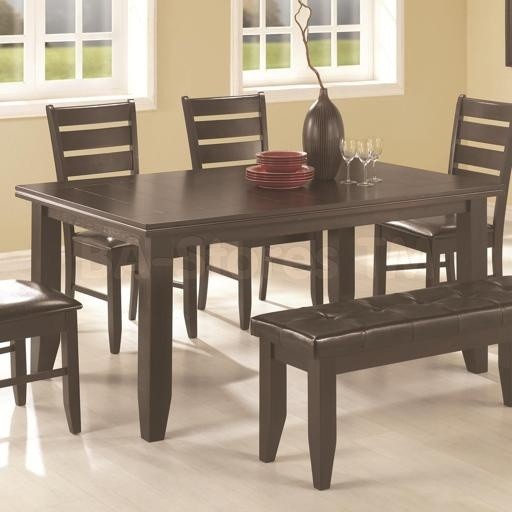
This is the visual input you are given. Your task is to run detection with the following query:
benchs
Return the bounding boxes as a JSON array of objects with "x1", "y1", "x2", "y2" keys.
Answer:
[{"x1": 249, "y1": 274, "x2": 512, "y2": 490}]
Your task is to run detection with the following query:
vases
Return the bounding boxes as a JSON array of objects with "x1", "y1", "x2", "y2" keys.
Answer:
[{"x1": 302, "y1": 88, "x2": 345, "y2": 180}]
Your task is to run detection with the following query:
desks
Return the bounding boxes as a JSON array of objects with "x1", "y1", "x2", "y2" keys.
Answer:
[{"x1": 14, "y1": 153, "x2": 505, "y2": 443}]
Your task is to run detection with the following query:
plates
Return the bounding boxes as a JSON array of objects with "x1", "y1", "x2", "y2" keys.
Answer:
[{"x1": 244, "y1": 151, "x2": 316, "y2": 192}]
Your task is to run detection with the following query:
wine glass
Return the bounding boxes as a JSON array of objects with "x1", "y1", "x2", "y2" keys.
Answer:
[
  {"x1": 367, "y1": 135, "x2": 386, "y2": 183},
  {"x1": 338, "y1": 137, "x2": 358, "y2": 185},
  {"x1": 354, "y1": 137, "x2": 375, "y2": 187}
]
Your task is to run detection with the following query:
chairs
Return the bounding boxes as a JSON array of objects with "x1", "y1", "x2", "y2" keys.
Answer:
[
  {"x1": 0, "y1": 279, "x2": 83, "y2": 434},
  {"x1": 182, "y1": 90, "x2": 324, "y2": 330},
  {"x1": 373, "y1": 94, "x2": 512, "y2": 298},
  {"x1": 46, "y1": 100, "x2": 198, "y2": 354}
]
